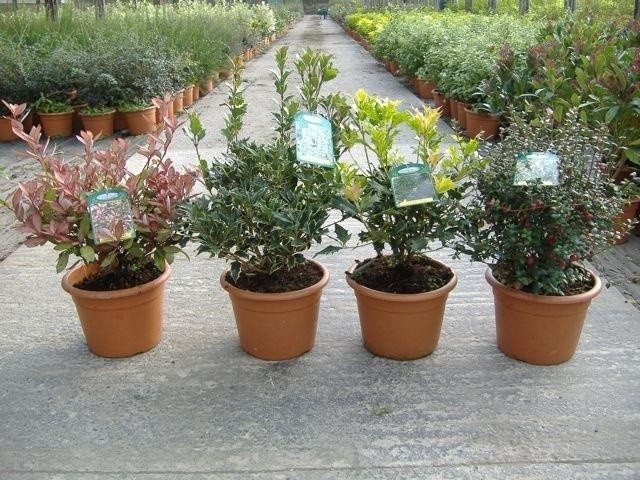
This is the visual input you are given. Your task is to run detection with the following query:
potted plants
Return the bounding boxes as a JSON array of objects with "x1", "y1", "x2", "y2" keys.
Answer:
[
  {"x1": 327, "y1": 83, "x2": 482, "y2": 362},
  {"x1": 442, "y1": 96, "x2": 639, "y2": 365},
  {"x1": 329, "y1": 0, "x2": 638, "y2": 245},
  {"x1": 167, "y1": 42, "x2": 359, "y2": 360},
  {"x1": 2, "y1": 94, "x2": 198, "y2": 358},
  {"x1": 0, "y1": 0, "x2": 302, "y2": 138}
]
